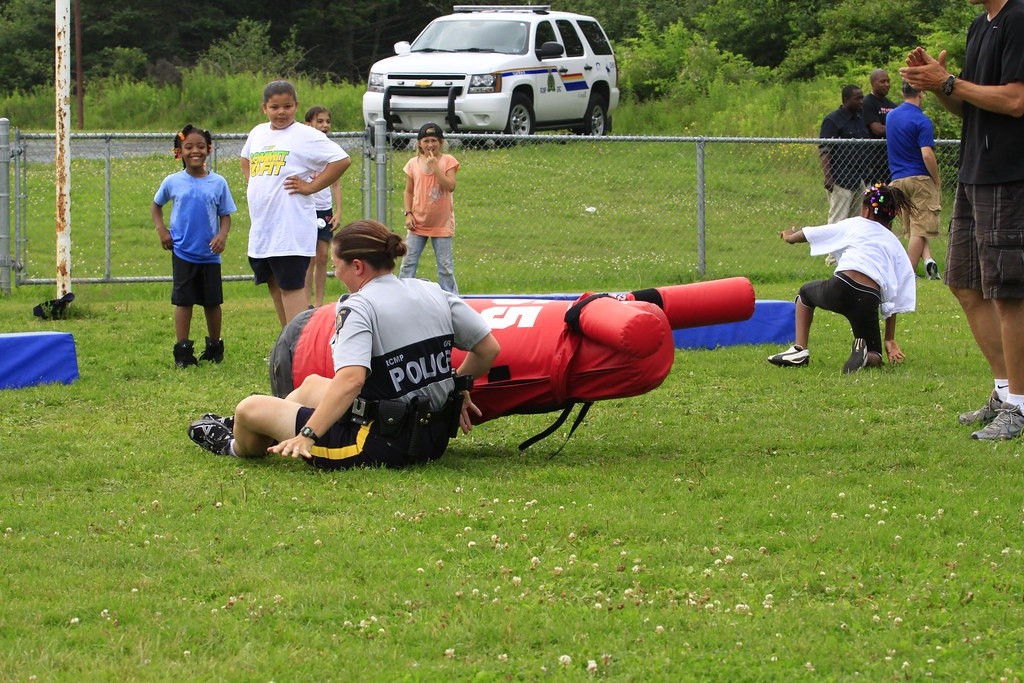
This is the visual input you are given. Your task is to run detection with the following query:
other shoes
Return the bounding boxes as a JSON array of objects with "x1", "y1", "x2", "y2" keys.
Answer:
[{"x1": 825, "y1": 255, "x2": 836, "y2": 267}]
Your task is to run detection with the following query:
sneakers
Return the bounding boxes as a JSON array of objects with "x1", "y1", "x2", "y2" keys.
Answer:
[
  {"x1": 200, "y1": 337, "x2": 224, "y2": 364},
  {"x1": 959, "y1": 389, "x2": 1004, "y2": 427},
  {"x1": 843, "y1": 338, "x2": 868, "y2": 374},
  {"x1": 189, "y1": 421, "x2": 234, "y2": 457},
  {"x1": 203, "y1": 413, "x2": 235, "y2": 429},
  {"x1": 173, "y1": 340, "x2": 198, "y2": 369},
  {"x1": 915, "y1": 274, "x2": 919, "y2": 279},
  {"x1": 971, "y1": 402, "x2": 1024, "y2": 442},
  {"x1": 767, "y1": 345, "x2": 810, "y2": 368},
  {"x1": 925, "y1": 259, "x2": 942, "y2": 280}
]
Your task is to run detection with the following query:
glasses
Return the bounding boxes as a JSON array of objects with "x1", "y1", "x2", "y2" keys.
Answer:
[{"x1": 922, "y1": 91, "x2": 927, "y2": 97}]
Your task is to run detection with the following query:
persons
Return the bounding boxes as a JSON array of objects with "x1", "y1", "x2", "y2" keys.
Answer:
[
  {"x1": 399, "y1": 122, "x2": 460, "y2": 295},
  {"x1": 769, "y1": 183, "x2": 917, "y2": 373},
  {"x1": 898, "y1": 0, "x2": 1024, "y2": 443},
  {"x1": 865, "y1": 70, "x2": 897, "y2": 231},
  {"x1": 819, "y1": 85, "x2": 865, "y2": 266},
  {"x1": 240, "y1": 81, "x2": 351, "y2": 328},
  {"x1": 305, "y1": 107, "x2": 341, "y2": 310},
  {"x1": 152, "y1": 125, "x2": 237, "y2": 368},
  {"x1": 187, "y1": 220, "x2": 500, "y2": 470},
  {"x1": 885, "y1": 80, "x2": 942, "y2": 280}
]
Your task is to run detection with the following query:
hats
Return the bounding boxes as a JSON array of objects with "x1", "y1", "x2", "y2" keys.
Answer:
[
  {"x1": 417, "y1": 123, "x2": 444, "y2": 140},
  {"x1": 32, "y1": 293, "x2": 75, "y2": 320}
]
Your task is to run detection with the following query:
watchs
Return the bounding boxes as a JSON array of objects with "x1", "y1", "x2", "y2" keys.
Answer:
[
  {"x1": 404, "y1": 211, "x2": 411, "y2": 215},
  {"x1": 941, "y1": 73, "x2": 958, "y2": 95},
  {"x1": 300, "y1": 426, "x2": 319, "y2": 442}
]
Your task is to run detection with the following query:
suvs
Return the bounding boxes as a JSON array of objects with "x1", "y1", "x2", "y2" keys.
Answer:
[{"x1": 363, "y1": 4, "x2": 621, "y2": 151}]
